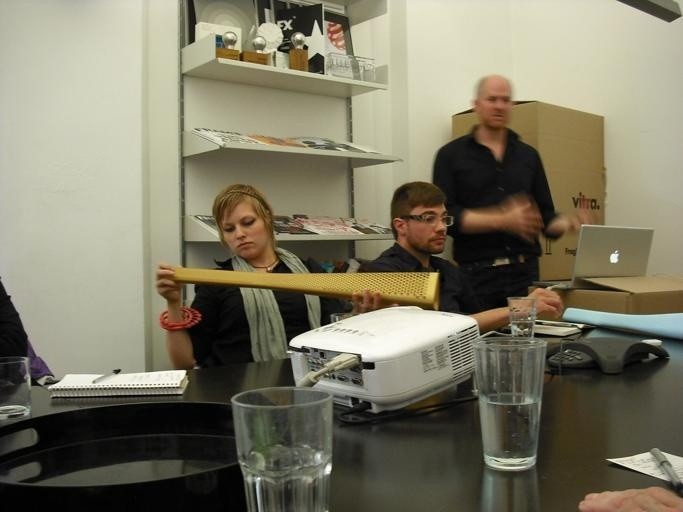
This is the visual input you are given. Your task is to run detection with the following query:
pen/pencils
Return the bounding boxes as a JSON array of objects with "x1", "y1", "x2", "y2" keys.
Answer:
[
  {"x1": 650, "y1": 446, "x2": 683, "y2": 489},
  {"x1": 535, "y1": 320, "x2": 579, "y2": 327},
  {"x1": 92, "y1": 368, "x2": 122, "y2": 384}
]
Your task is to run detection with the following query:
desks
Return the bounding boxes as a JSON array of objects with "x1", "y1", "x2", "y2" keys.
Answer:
[{"x1": 0, "y1": 327, "x2": 683, "y2": 512}]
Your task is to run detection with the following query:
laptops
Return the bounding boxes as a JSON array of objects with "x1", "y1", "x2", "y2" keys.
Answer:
[{"x1": 532, "y1": 225, "x2": 655, "y2": 290}]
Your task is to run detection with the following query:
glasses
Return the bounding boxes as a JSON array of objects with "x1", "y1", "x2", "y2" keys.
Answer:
[{"x1": 401, "y1": 213, "x2": 452, "y2": 227}]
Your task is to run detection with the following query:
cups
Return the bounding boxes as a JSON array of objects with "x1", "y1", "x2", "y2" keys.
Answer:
[
  {"x1": 0, "y1": 357, "x2": 31, "y2": 421},
  {"x1": 231, "y1": 387, "x2": 333, "y2": 512},
  {"x1": 474, "y1": 338, "x2": 547, "y2": 471},
  {"x1": 507, "y1": 297, "x2": 536, "y2": 338}
]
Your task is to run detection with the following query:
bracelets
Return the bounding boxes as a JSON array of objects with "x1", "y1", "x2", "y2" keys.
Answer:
[{"x1": 160, "y1": 306, "x2": 202, "y2": 330}]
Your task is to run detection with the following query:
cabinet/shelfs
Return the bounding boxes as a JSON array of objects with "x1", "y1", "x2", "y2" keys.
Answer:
[{"x1": 151, "y1": 0, "x2": 404, "y2": 371}]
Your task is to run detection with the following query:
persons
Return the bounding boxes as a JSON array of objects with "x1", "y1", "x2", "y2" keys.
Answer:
[
  {"x1": 352, "y1": 182, "x2": 563, "y2": 333},
  {"x1": 1, "y1": 274, "x2": 56, "y2": 386},
  {"x1": 155, "y1": 183, "x2": 399, "y2": 368},
  {"x1": 433, "y1": 74, "x2": 591, "y2": 312},
  {"x1": 579, "y1": 486, "x2": 683, "y2": 512}
]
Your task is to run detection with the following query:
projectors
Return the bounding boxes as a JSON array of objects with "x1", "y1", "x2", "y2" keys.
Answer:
[{"x1": 286, "y1": 304, "x2": 479, "y2": 414}]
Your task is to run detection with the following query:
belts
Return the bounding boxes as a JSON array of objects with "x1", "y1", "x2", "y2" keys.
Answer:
[{"x1": 469, "y1": 252, "x2": 527, "y2": 267}]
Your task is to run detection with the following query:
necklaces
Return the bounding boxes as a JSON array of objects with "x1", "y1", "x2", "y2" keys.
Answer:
[{"x1": 253, "y1": 258, "x2": 279, "y2": 272}]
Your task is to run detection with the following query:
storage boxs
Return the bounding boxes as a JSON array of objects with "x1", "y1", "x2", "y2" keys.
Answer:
[
  {"x1": 527, "y1": 274, "x2": 683, "y2": 322},
  {"x1": 451, "y1": 100, "x2": 604, "y2": 281}
]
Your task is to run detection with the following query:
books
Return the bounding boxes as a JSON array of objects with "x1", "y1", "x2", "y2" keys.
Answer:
[
  {"x1": 194, "y1": 210, "x2": 395, "y2": 237},
  {"x1": 194, "y1": 124, "x2": 392, "y2": 155}
]
